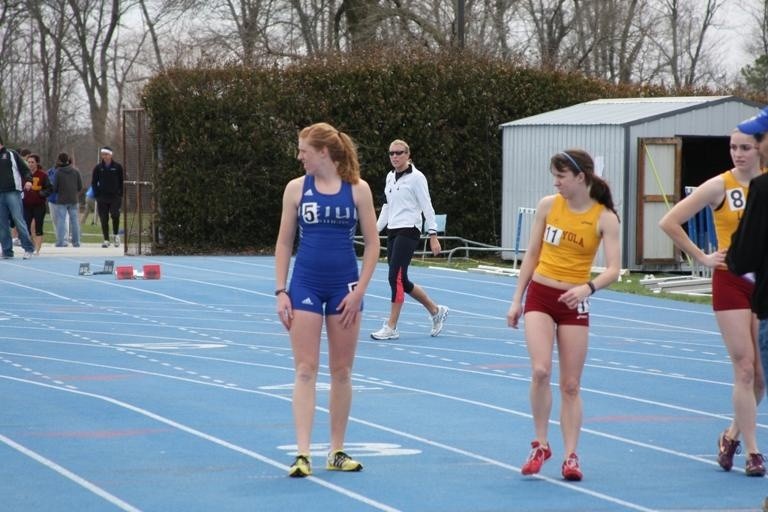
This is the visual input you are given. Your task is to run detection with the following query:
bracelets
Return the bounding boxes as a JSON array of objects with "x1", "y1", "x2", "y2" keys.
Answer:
[
  {"x1": 274, "y1": 288, "x2": 286, "y2": 296},
  {"x1": 586, "y1": 282, "x2": 596, "y2": 295}
]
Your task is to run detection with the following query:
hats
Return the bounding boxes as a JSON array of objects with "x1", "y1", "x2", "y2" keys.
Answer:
[
  {"x1": 737, "y1": 107, "x2": 768, "y2": 135},
  {"x1": 101, "y1": 146, "x2": 112, "y2": 155}
]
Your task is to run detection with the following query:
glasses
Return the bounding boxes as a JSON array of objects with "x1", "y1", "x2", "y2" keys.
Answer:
[{"x1": 390, "y1": 151, "x2": 405, "y2": 155}]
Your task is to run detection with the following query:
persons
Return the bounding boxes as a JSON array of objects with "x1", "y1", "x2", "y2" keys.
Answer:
[
  {"x1": 507, "y1": 150, "x2": 622, "y2": 481},
  {"x1": 275, "y1": 123, "x2": 379, "y2": 478},
  {"x1": 371, "y1": 138, "x2": 448, "y2": 341},
  {"x1": 0, "y1": 137, "x2": 123, "y2": 259},
  {"x1": 658, "y1": 127, "x2": 765, "y2": 476},
  {"x1": 724, "y1": 108, "x2": 768, "y2": 511}
]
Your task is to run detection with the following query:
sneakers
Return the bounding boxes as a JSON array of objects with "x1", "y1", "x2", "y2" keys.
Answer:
[
  {"x1": 427, "y1": 304, "x2": 449, "y2": 337},
  {"x1": 562, "y1": 452, "x2": 583, "y2": 481},
  {"x1": 521, "y1": 441, "x2": 552, "y2": 476},
  {"x1": 1, "y1": 237, "x2": 42, "y2": 260},
  {"x1": 370, "y1": 324, "x2": 400, "y2": 341},
  {"x1": 290, "y1": 454, "x2": 312, "y2": 477},
  {"x1": 745, "y1": 453, "x2": 767, "y2": 477},
  {"x1": 325, "y1": 450, "x2": 363, "y2": 471},
  {"x1": 101, "y1": 235, "x2": 121, "y2": 248},
  {"x1": 717, "y1": 428, "x2": 742, "y2": 471}
]
